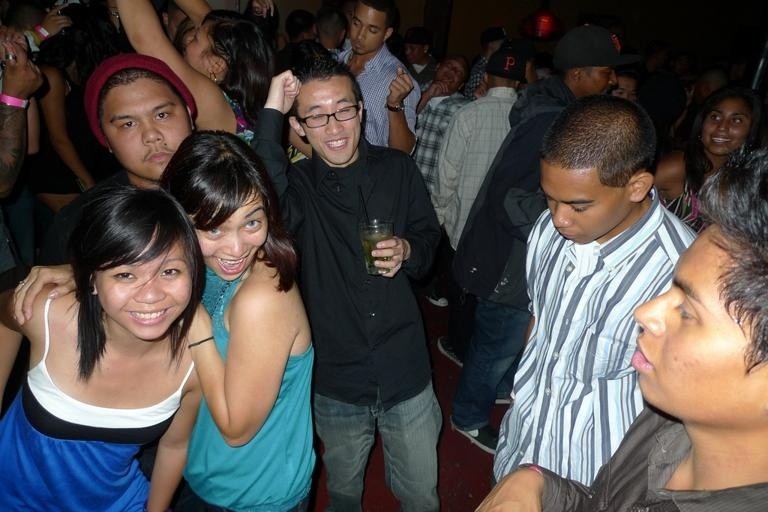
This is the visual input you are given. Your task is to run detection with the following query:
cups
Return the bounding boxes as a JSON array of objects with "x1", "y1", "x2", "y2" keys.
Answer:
[{"x1": 359, "y1": 219, "x2": 395, "y2": 277}]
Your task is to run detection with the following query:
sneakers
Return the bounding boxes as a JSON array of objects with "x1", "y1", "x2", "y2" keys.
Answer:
[
  {"x1": 447, "y1": 413, "x2": 501, "y2": 456},
  {"x1": 493, "y1": 377, "x2": 513, "y2": 405},
  {"x1": 436, "y1": 334, "x2": 465, "y2": 367}
]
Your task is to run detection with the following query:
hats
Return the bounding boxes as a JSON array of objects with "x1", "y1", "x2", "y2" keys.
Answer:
[
  {"x1": 401, "y1": 25, "x2": 433, "y2": 45},
  {"x1": 479, "y1": 26, "x2": 512, "y2": 44},
  {"x1": 550, "y1": 23, "x2": 624, "y2": 72},
  {"x1": 83, "y1": 52, "x2": 198, "y2": 150},
  {"x1": 484, "y1": 49, "x2": 528, "y2": 84}
]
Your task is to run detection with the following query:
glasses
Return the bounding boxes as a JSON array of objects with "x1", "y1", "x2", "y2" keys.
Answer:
[{"x1": 297, "y1": 104, "x2": 362, "y2": 129}]
